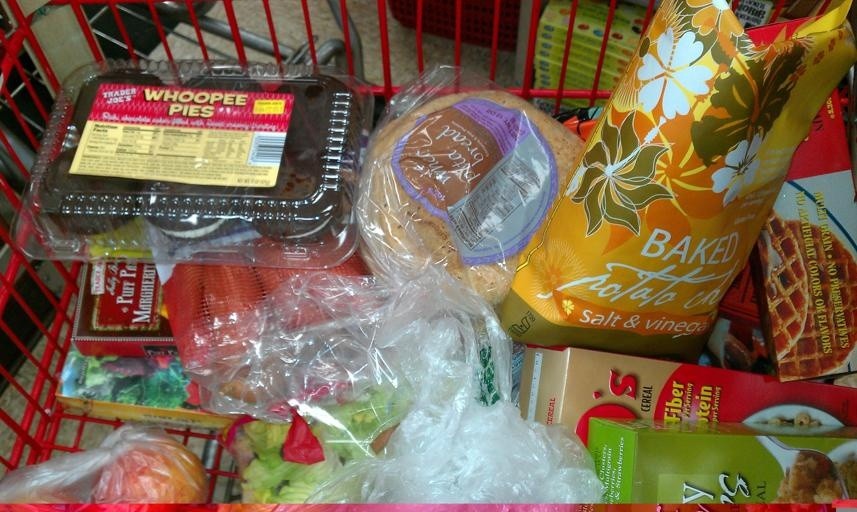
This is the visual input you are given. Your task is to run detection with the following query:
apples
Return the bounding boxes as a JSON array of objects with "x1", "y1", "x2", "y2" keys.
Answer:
[
  {"x1": 12, "y1": 487, "x2": 84, "y2": 504},
  {"x1": 89, "y1": 436, "x2": 212, "y2": 504}
]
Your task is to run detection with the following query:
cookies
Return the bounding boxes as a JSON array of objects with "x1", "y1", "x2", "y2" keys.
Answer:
[
  {"x1": 779, "y1": 217, "x2": 857, "y2": 382},
  {"x1": 36, "y1": 69, "x2": 363, "y2": 246},
  {"x1": 755, "y1": 205, "x2": 809, "y2": 363}
]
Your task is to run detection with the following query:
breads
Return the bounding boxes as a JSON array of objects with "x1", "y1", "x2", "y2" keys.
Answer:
[{"x1": 355, "y1": 88, "x2": 589, "y2": 311}]
